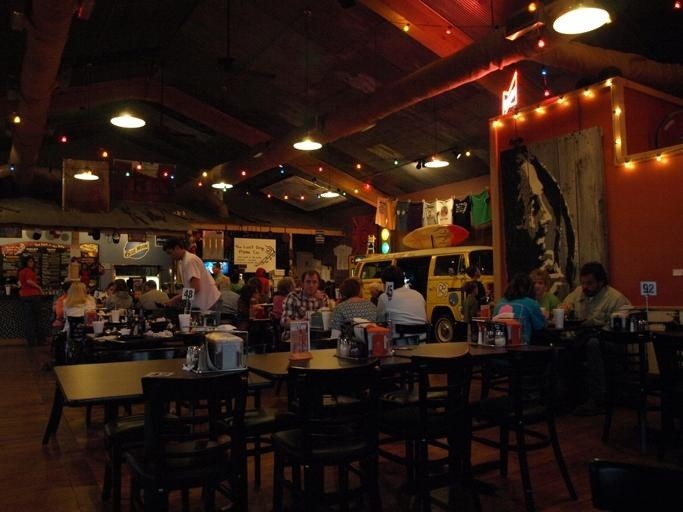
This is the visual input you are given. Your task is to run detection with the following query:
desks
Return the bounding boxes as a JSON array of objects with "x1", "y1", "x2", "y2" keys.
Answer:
[
  {"x1": 645, "y1": 329, "x2": 681, "y2": 353},
  {"x1": 393, "y1": 341, "x2": 568, "y2": 396},
  {"x1": 244, "y1": 347, "x2": 412, "y2": 425},
  {"x1": 536, "y1": 319, "x2": 596, "y2": 342},
  {"x1": 284, "y1": 336, "x2": 339, "y2": 348},
  {"x1": 42, "y1": 357, "x2": 269, "y2": 487}
]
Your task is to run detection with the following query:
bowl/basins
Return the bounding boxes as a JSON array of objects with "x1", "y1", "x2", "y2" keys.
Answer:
[{"x1": 154, "y1": 302, "x2": 173, "y2": 309}]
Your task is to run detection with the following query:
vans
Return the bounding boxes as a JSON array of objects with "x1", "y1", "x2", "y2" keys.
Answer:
[{"x1": 353, "y1": 246, "x2": 494, "y2": 343}]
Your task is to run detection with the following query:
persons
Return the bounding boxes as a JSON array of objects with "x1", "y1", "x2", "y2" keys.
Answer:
[
  {"x1": 17, "y1": 255, "x2": 50, "y2": 346},
  {"x1": 461, "y1": 262, "x2": 632, "y2": 416}
]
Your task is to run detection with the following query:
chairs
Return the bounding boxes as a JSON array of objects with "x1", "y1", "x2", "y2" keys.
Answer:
[
  {"x1": 270, "y1": 359, "x2": 382, "y2": 510},
  {"x1": 471, "y1": 350, "x2": 579, "y2": 508},
  {"x1": 393, "y1": 323, "x2": 431, "y2": 344},
  {"x1": 67, "y1": 313, "x2": 239, "y2": 357},
  {"x1": 382, "y1": 349, "x2": 470, "y2": 510},
  {"x1": 250, "y1": 318, "x2": 277, "y2": 347},
  {"x1": 598, "y1": 325, "x2": 655, "y2": 456},
  {"x1": 123, "y1": 368, "x2": 250, "y2": 510}
]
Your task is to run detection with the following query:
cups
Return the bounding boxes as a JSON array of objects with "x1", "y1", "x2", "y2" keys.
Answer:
[
  {"x1": 552, "y1": 309, "x2": 564, "y2": 328},
  {"x1": 5, "y1": 287, "x2": 10, "y2": 295}
]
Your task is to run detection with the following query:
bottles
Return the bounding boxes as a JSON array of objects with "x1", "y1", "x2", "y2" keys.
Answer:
[
  {"x1": 186, "y1": 344, "x2": 209, "y2": 371},
  {"x1": 337, "y1": 336, "x2": 366, "y2": 357},
  {"x1": 92, "y1": 309, "x2": 208, "y2": 338},
  {"x1": 629, "y1": 318, "x2": 648, "y2": 333},
  {"x1": 477, "y1": 327, "x2": 505, "y2": 347}
]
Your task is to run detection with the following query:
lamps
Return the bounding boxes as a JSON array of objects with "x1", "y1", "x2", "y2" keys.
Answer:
[
  {"x1": 293, "y1": 129, "x2": 323, "y2": 151},
  {"x1": 73, "y1": 167, "x2": 98, "y2": 181},
  {"x1": 211, "y1": 177, "x2": 233, "y2": 190},
  {"x1": 424, "y1": 154, "x2": 450, "y2": 168},
  {"x1": 110, "y1": 106, "x2": 146, "y2": 129},
  {"x1": 320, "y1": 190, "x2": 339, "y2": 198},
  {"x1": 552, "y1": 2, "x2": 611, "y2": 34}
]
